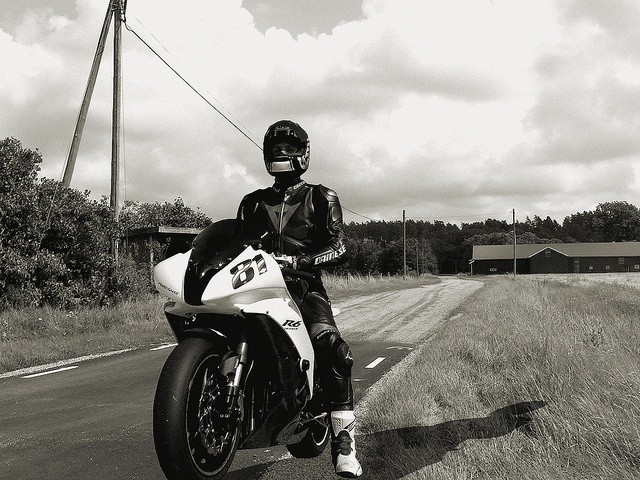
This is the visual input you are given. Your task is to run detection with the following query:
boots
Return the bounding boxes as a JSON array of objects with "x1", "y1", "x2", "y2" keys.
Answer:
[{"x1": 329, "y1": 410, "x2": 362, "y2": 478}]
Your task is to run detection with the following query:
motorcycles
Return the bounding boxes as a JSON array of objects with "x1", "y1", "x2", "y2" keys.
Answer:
[{"x1": 150, "y1": 219, "x2": 333, "y2": 480}]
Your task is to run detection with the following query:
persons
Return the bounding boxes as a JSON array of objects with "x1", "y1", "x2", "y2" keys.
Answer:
[{"x1": 234, "y1": 120, "x2": 364, "y2": 478}]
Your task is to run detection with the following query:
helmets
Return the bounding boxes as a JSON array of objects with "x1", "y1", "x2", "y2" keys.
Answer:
[{"x1": 263, "y1": 120, "x2": 310, "y2": 175}]
